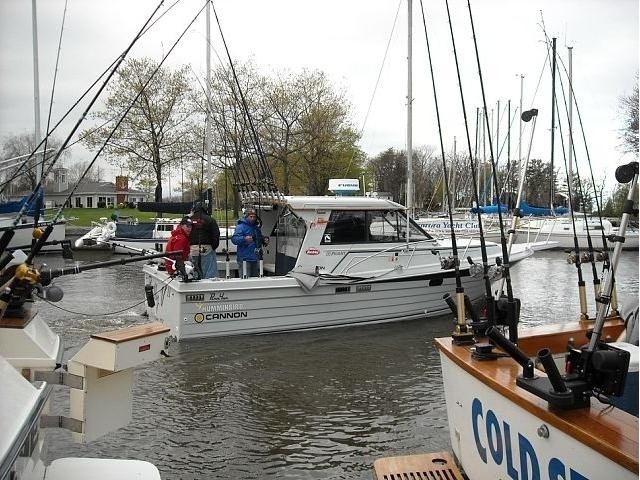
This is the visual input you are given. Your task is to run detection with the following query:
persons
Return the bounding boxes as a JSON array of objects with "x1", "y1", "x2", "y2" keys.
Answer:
[
  {"x1": 189, "y1": 209, "x2": 220, "y2": 278},
  {"x1": 165, "y1": 216, "x2": 200, "y2": 281},
  {"x1": 231, "y1": 207, "x2": 269, "y2": 278}
]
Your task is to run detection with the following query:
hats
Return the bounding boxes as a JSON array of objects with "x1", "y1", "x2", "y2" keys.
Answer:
[
  {"x1": 192, "y1": 202, "x2": 209, "y2": 214},
  {"x1": 244, "y1": 207, "x2": 257, "y2": 219},
  {"x1": 180, "y1": 216, "x2": 197, "y2": 225}
]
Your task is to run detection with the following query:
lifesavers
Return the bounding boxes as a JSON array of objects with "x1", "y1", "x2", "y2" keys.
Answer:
[{"x1": 106, "y1": 222, "x2": 117, "y2": 234}]
